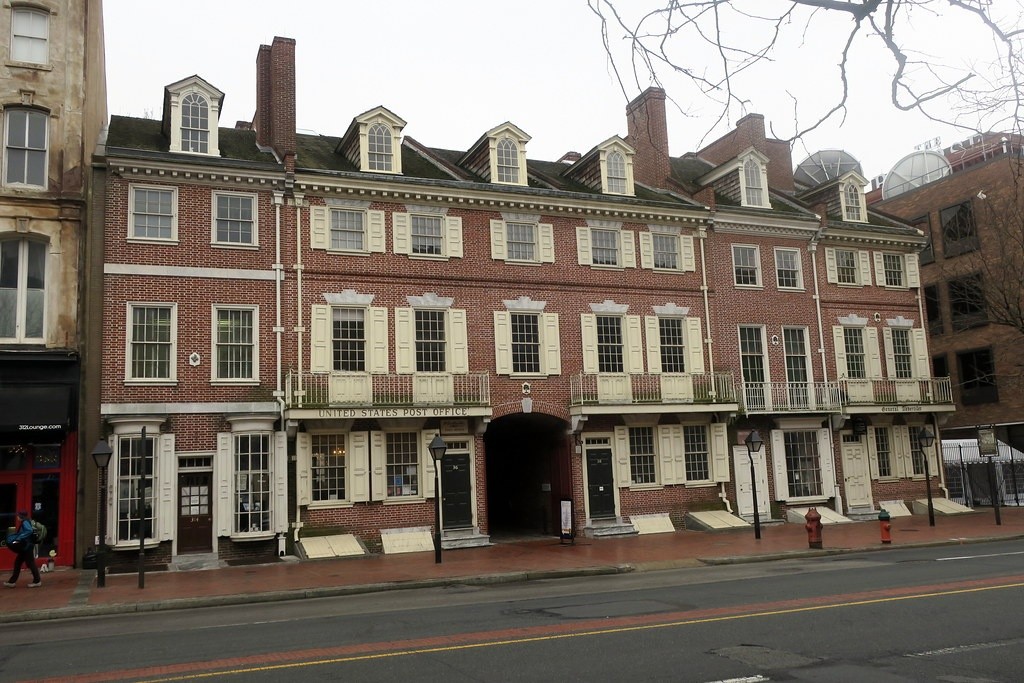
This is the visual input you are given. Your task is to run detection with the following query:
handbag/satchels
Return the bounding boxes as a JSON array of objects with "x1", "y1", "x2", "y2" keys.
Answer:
[{"x1": 6, "y1": 533, "x2": 28, "y2": 552}]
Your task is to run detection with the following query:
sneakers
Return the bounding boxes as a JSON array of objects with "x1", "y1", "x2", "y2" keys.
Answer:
[
  {"x1": 27, "y1": 581, "x2": 42, "y2": 587},
  {"x1": 3, "y1": 582, "x2": 16, "y2": 588}
]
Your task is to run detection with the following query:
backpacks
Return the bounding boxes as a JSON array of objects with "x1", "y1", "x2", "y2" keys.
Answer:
[{"x1": 26, "y1": 519, "x2": 47, "y2": 544}]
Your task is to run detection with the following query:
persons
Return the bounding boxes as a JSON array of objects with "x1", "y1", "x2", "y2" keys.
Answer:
[{"x1": 3, "y1": 510, "x2": 41, "y2": 588}]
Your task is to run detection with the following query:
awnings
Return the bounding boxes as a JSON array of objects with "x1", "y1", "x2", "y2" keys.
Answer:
[{"x1": 0, "y1": 386, "x2": 77, "y2": 434}]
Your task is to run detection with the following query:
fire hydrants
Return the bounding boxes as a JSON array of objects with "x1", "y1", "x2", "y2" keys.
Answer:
[
  {"x1": 804, "y1": 506, "x2": 824, "y2": 550},
  {"x1": 877, "y1": 508, "x2": 892, "y2": 545}
]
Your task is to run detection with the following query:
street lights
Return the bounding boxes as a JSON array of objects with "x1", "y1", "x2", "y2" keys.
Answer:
[
  {"x1": 427, "y1": 432, "x2": 447, "y2": 564},
  {"x1": 742, "y1": 429, "x2": 768, "y2": 534},
  {"x1": 919, "y1": 426, "x2": 941, "y2": 526},
  {"x1": 90, "y1": 435, "x2": 117, "y2": 591}
]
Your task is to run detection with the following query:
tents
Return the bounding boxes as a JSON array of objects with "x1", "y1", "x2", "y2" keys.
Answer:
[{"x1": 942, "y1": 438, "x2": 1024, "y2": 506}]
